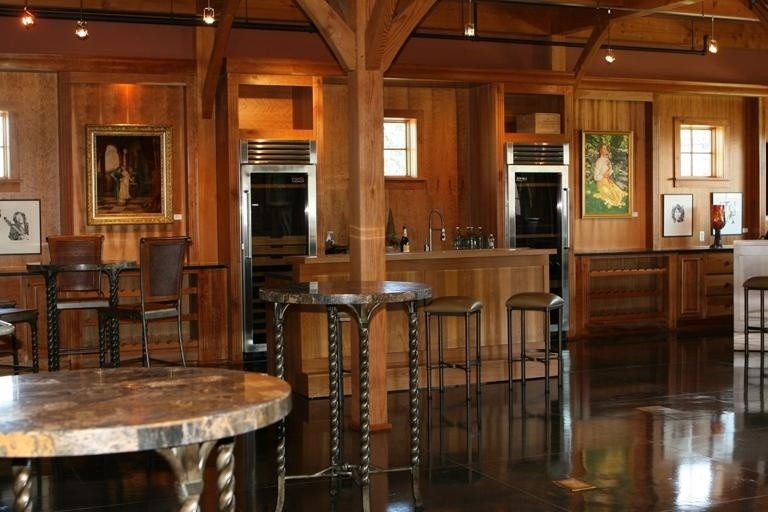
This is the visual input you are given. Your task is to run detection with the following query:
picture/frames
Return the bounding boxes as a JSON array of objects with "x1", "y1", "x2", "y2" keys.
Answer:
[
  {"x1": 0, "y1": 199, "x2": 42, "y2": 255},
  {"x1": 712, "y1": 192, "x2": 743, "y2": 235},
  {"x1": 581, "y1": 130, "x2": 633, "y2": 218},
  {"x1": 86, "y1": 123, "x2": 173, "y2": 225},
  {"x1": 663, "y1": 194, "x2": 693, "y2": 237}
]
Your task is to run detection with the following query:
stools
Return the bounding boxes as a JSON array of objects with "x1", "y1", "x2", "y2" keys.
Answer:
[
  {"x1": 424, "y1": 296, "x2": 483, "y2": 400},
  {"x1": 743, "y1": 276, "x2": 768, "y2": 356},
  {"x1": 505, "y1": 292, "x2": 564, "y2": 393}
]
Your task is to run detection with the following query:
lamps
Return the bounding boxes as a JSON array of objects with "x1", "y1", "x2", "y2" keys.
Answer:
[
  {"x1": 21, "y1": 0, "x2": 36, "y2": 33},
  {"x1": 464, "y1": 0, "x2": 474, "y2": 36},
  {"x1": 605, "y1": 8, "x2": 616, "y2": 63},
  {"x1": 74, "y1": 0, "x2": 89, "y2": 40},
  {"x1": 203, "y1": 0, "x2": 215, "y2": 24},
  {"x1": 708, "y1": 5, "x2": 718, "y2": 53}
]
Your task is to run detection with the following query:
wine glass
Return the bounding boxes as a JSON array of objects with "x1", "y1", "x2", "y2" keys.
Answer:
[{"x1": 708, "y1": 205, "x2": 727, "y2": 250}]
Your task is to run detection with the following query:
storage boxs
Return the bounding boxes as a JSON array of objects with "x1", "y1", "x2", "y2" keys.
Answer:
[{"x1": 516, "y1": 113, "x2": 561, "y2": 134}]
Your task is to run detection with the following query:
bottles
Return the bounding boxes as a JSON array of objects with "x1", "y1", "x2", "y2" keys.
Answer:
[
  {"x1": 451, "y1": 226, "x2": 485, "y2": 249},
  {"x1": 400, "y1": 225, "x2": 409, "y2": 253},
  {"x1": 488, "y1": 234, "x2": 495, "y2": 249}
]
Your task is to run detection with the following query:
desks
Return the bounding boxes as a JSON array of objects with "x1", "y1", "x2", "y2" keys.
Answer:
[
  {"x1": 0, "y1": 368, "x2": 292, "y2": 512},
  {"x1": 259, "y1": 280, "x2": 432, "y2": 512}
]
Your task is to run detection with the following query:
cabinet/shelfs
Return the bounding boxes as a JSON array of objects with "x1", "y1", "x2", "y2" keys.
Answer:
[
  {"x1": 678, "y1": 253, "x2": 734, "y2": 324},
  {"x1": 576, "y1": 253, "x2": 678, "y2": 337}
]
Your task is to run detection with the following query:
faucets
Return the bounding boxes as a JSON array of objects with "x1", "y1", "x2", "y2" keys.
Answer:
[
  {"x1": 325, "y1": 230, "x2": 335, "y2": 246},
  {"x1": 423, "y1": 209, "x2": 446, "y2": 252}
]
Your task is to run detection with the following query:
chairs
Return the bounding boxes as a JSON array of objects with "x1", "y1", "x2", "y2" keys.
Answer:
[{"x1": 0, "y1": 234, "x2": 192, "y2": 367}]
